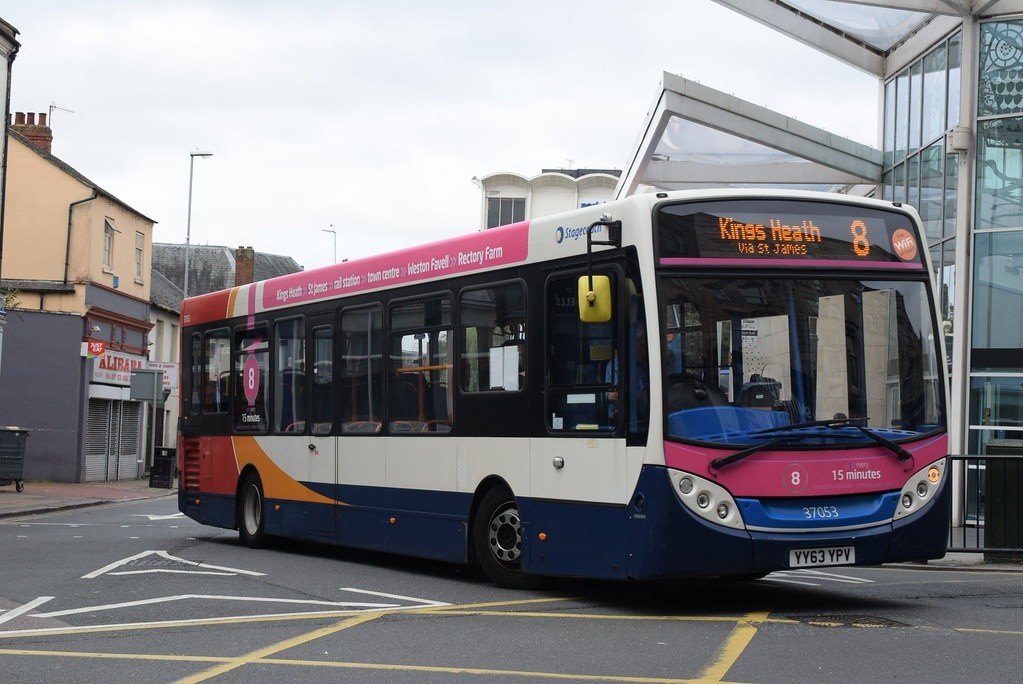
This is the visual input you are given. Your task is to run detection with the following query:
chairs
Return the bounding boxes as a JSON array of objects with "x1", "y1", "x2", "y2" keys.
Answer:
[
  {"x1": 216, "y1": 368, "x2": 453, "y2": 432},
  {"x1": 596, "y1": 357, "x2": 605, "y2": 410}
]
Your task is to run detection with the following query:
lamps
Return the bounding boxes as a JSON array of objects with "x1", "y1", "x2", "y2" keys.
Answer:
[
  {"x1": 89, "y1": 321, "x2": 101, "y2": 334},
  {"x1": 945, "y1": 128, "x2": 971, "y2": 154},
  {"x1": 145, "y1": 339, "x2": 154, "y2": 346}
]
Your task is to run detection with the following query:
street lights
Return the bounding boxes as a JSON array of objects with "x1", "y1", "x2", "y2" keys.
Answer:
[
  {"x1": 184, "y1": 152, "x2": 214, "y2": 301},
  {"x1": 324, "y1": 227, "x2": 338, "y2": 263}
]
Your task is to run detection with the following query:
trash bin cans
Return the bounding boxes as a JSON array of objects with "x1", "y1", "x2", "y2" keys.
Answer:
[
  {"x1": 154, "y1": 447, "x2": 176, "y2": 488},
  {"x1": 984, "y1": 438, "x2": 1023, "y2": 560},
  {"x1": 0, "y1": 425, "x2": 33, "y2": 492}
]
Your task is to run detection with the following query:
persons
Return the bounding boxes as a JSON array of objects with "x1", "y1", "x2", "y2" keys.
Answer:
[
  {"x1": 605, "y1": 355, "x2": 619, "y2": 427},
  {"x1": 501, "y1": 338, "x2": 526, "y2": 390}
]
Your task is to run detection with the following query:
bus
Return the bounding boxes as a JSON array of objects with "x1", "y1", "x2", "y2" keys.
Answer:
[{"x1": 176, "y1": 187, "x2": 950, "y2": 592}]
